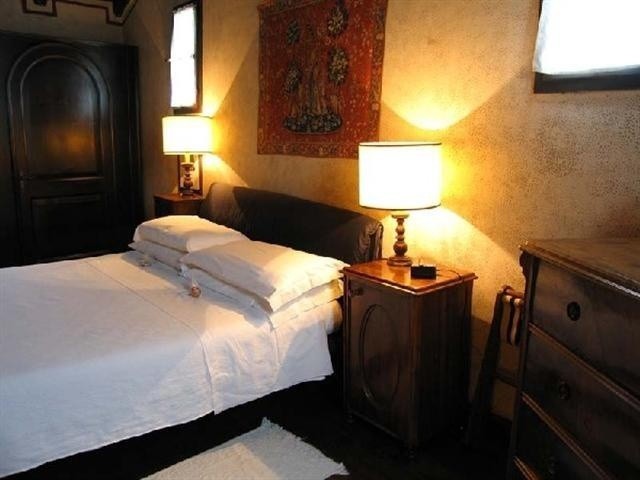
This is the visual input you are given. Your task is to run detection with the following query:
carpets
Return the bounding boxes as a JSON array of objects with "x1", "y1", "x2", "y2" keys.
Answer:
[{"x1": 138, "y1": 416, "x2": 350, "y2": 480}]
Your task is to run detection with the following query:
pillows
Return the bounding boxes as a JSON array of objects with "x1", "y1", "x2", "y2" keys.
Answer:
[{"x1": 128, "y1": 215, "x2": 351, "y2": 329}]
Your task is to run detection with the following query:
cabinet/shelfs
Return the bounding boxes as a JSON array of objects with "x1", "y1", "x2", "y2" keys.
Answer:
[{"x1": 503, "y1": 237, "x2": 639, "y2": 480}]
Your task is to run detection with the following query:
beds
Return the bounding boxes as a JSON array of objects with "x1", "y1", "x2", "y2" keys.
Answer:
[{"x1": 0, "y1": 183, "x2": 384, "y2": 480}]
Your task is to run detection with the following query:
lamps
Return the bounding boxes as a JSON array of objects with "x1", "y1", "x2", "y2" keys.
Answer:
[
  {"x1": 358, "y1": 142, "x2": 442, "y2": 265},
  {"x1": 162, "y1": 115, "x2": 213, "y2": 198}
]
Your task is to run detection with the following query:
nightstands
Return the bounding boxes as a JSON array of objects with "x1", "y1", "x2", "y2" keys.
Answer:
[
  {"x1": 153, "y1": 193, "x2": 204, "y2": 218},
  {"x1": 340, "y1": 258, "x2": 478, "y2": 460}
]
